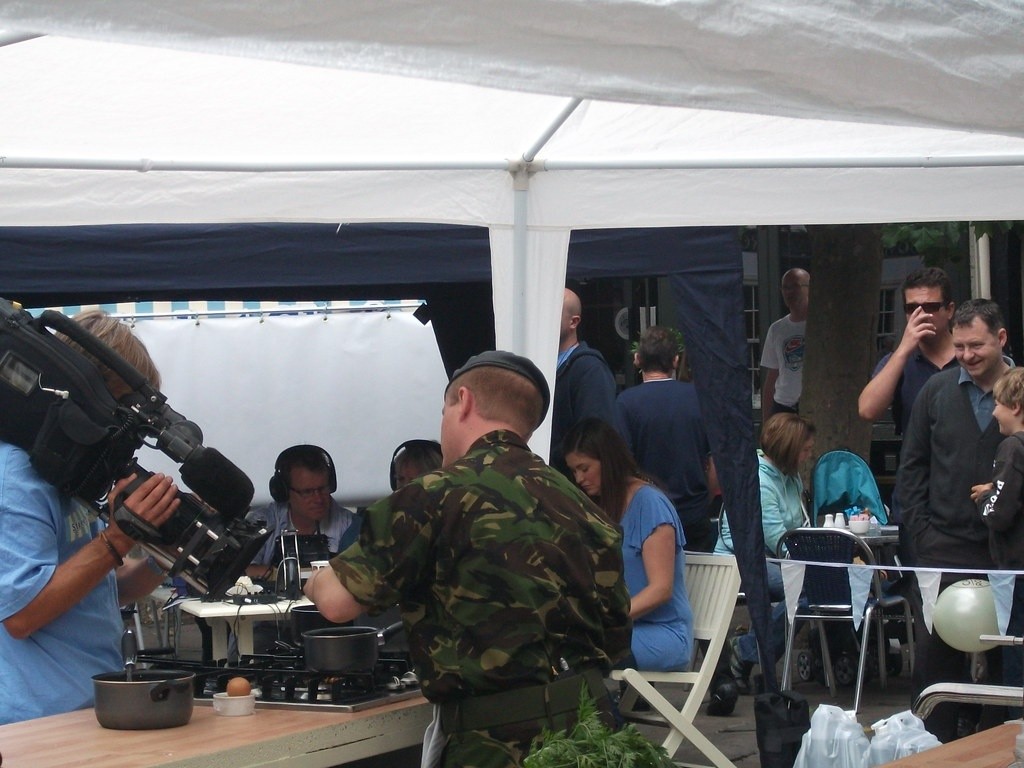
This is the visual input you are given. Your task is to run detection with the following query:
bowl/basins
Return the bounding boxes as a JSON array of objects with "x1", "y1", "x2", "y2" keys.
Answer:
[{"x1": 848, "y1": 521, "x2": 869, "y2": 533}]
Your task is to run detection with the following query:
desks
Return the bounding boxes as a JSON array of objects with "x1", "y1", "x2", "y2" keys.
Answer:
[
  {"x1": 150, "y1": 586, "x2": 317, "y2": 663},
  {"x1": 858, "y1": 531, "x2": 899, "y2": 549},
  {"x1": 0, "y1": 693, "x2": 435, "y2": 768}
]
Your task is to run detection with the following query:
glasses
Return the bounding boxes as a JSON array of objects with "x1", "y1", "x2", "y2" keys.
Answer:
[
  {"x1": 287, "y1": 485, "x2": 332, "y2": 497},
  {"x1": 903, "y1": 301, "x2": 947, "y2": 314}
]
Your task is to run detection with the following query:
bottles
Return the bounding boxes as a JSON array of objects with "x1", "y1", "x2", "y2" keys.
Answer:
[
  {"x1": 834, "y1": 513, "x2": 846, "y2": 528},
  {"x1": 822, "y1": 514, "x2": 834, "y2": 530}
]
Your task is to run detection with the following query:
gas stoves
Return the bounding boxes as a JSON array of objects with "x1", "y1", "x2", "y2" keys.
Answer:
[{"x1": 132, "y1": 631, "x2": 432, "y2": 713}]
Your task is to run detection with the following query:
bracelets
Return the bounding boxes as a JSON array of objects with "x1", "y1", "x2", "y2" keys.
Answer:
[{"x1": 99, "y1": 531, "x2": 123, "y2": 567}]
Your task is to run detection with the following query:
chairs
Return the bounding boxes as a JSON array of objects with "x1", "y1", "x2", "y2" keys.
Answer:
[{"x1": 610, "y1": 525, "x2": 1024, "y2": 768}]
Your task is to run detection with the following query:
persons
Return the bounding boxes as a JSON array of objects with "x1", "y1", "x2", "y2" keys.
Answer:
[
  {"x1": 390, "y1": 440, "x2": 443, "y2": 492},
  {"x1": 0, "y1": 310, "x2": 216, "y2": 725},
  {"x1": 617, "y1": 327, "x2": 738, "y2": 716},
  {"x1": 548, "y1": 288, "x2": 618, "y2": 504},
  {"x1": 303, "y1": 350, "x2": 638, "y2": 768},
  {"x1": 228, "y1": 446, "x2": 362, "y2": 665},
  {"x1": 713, "y1": 268, "x2": 1024, "y2": 744},
  {"x1": 561, "y1": 415, "x2": 693, "y2": 730}
]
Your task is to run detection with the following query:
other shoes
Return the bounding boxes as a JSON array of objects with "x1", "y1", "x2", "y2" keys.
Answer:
[{"x1": 708, "y1": 684, "x2": 737, "y2": 715}]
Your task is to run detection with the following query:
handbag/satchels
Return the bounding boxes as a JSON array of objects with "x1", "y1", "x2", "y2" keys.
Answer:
[
  {"x1": 793, "y1": 704, "x2": 943, "y2": 768},
  {"x1": 754, "y1": 689, "x2": 810, "y2": 768}
]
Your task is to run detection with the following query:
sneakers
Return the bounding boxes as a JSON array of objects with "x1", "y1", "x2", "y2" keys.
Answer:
[{"x1": 730, "y1": 636, "x2": 752, "y2": 692}]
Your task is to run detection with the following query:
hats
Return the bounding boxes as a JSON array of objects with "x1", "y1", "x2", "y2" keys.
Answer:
[{"x1": 444, "y1": 349, "x2": 550, "y2": 430}]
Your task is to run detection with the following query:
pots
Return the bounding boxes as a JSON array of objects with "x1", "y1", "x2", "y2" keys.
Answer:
[
  {"x1": 300, "y1": 617, "x2": 405, "y2": 675},
  {"x1": 91, "y1": 667, "x2": 196, "y2": 729}
]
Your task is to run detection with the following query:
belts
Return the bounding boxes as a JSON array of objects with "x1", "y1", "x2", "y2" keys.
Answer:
[{"x1": 440, "y1": 667, "x2": 608, "y2": 735}]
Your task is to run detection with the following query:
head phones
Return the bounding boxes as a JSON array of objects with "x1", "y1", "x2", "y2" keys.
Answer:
[
  {"x1": 389, "y1": 439, "x2": 443, "y2": 492},
  {"x1": 268, "y1": 444, "x2": 337, "y2": 503}
]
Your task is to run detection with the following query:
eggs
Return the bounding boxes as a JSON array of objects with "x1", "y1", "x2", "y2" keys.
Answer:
[{"x1": 226, "y1": 677, "x2": 251, "y2": 696}]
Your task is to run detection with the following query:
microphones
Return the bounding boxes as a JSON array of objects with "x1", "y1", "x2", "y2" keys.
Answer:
[{"x1": 157, "y1": 430, "x2": 255, "y2": 514}]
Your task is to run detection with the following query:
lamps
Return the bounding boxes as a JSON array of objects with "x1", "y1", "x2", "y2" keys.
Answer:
[{"x1": 414, "y1": 302, "x2": 433, "y2": 325}]
